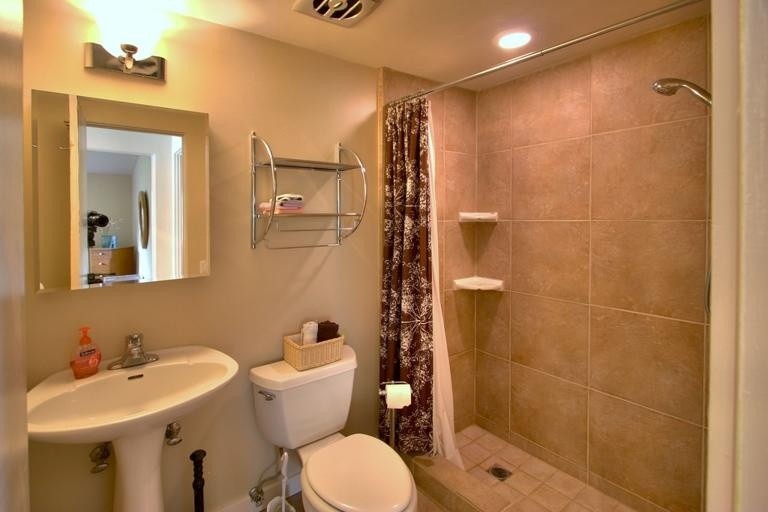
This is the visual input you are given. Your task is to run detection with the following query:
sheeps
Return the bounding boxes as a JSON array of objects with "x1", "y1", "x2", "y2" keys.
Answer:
[{"x1": 107, "y1": 332, "x2": 159, "y2": 370}]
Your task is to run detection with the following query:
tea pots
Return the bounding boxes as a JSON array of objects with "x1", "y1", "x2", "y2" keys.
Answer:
[{"x1": 79, "y1": 122, "x2": 183, "y2": 288}]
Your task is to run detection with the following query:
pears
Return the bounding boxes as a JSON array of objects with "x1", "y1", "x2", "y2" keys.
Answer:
[{"x1": 69, "y1": 327, "x2": 102, "y2": 380}]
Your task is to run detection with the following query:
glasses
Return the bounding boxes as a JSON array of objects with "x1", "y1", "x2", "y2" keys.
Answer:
[{"x1": 495, "y1": 29, "x2": 535, "y2": 49}]
